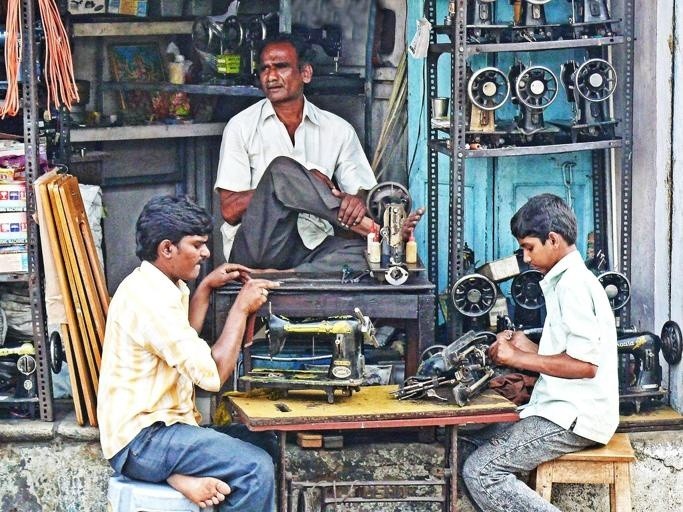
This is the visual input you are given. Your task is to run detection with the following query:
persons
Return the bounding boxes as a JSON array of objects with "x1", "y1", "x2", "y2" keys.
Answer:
[
  {"x1": 213, "y1": 34, "x2": 426, "y2": 271},
  {"x1": 96, "y1": 194, "x2": 276, "y2": 512},
  {"x1": 462, "y1": 193, "x2": 620, "y2": 512}
]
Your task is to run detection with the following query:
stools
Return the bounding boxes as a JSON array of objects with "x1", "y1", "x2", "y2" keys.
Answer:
[
  {"x1": 534, "y1": 431, "x2": 636, "y2": 509},
  {"x1": 103, "y1": 474, "x2": 215, "y2": 512}
]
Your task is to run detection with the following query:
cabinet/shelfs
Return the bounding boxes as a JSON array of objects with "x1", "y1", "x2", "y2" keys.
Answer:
[{"x1": 428, "y1": 2, "x2": 632, "y2": 345}]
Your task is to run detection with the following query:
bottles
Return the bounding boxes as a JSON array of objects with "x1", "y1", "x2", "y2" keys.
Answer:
[
  {"x1": 405, "y1": 226, "x2": 417, "y2": 265},
  {"x1": 367, "y1": 220, "x2": 381, "y2": 265}
]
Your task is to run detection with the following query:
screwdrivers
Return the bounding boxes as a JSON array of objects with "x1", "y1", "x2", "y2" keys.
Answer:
[{"x1": 340, "y1": 264, "x2": 362, "y2": 284}]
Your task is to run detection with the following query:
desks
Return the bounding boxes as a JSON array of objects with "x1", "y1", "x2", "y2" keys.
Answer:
[
  {"x1": 228, "y1": 382, "x2": 520, "y2": 511},
  {"x1": 213, "y1": 269, "x2": 438, "y2": 380}
]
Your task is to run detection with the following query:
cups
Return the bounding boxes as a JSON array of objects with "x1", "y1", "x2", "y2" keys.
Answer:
[{"x1": 433, "y1": 96, "x2": 449, "y2": 121}]
